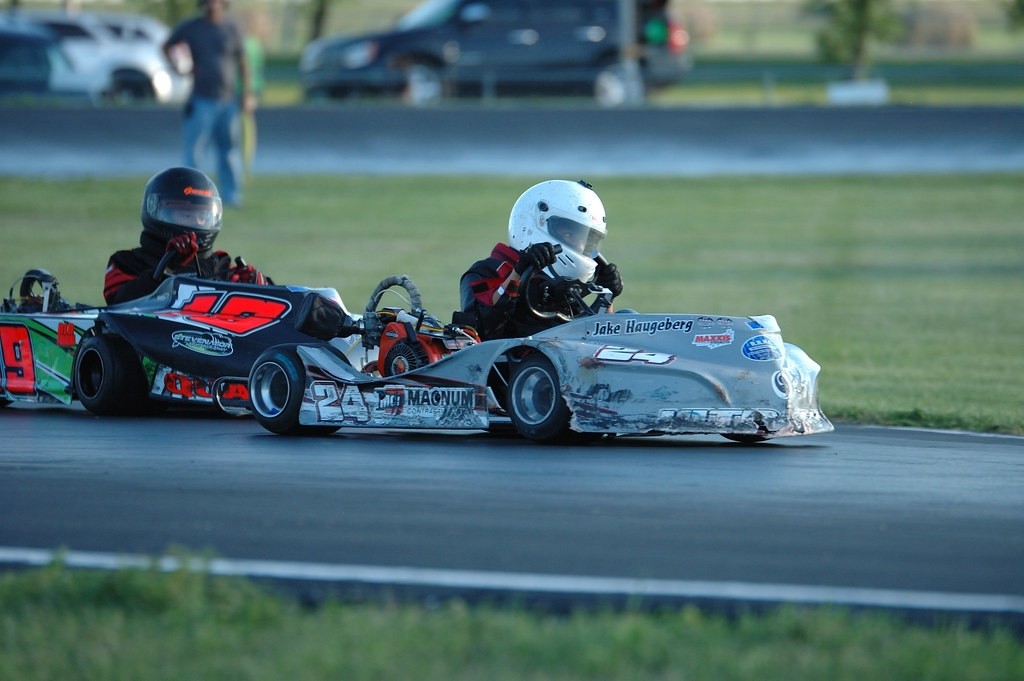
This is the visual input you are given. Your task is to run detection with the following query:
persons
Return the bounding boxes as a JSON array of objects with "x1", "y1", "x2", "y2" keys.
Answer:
[
  {"x1": 163, "y1": 0, "x2": 257, "y2": 210},
  {"x1": 460, "y1": 180, "x2": 623, "y2": 344},
  {"x1": 104, "y1": 166, "x2": 267, "y2": 307}
]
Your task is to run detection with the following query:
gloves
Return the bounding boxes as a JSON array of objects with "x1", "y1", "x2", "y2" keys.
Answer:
[
  {"x1": 595, "y1": 263, "x2": 623, "y2": 300},
  {"x1": 520, "y1": 242, "x2": 556, "y2": 270},
  {"x1": 229, "y1": 264, "x2": 257, "y2": 287},
  {"x1": 165, "y1": 231, "x2": 199, "y2": 269}
]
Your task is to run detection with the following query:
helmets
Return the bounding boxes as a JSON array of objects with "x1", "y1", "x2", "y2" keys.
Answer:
[
  {"x1": 140, "y1": 166, "x2": 223, "y2": 252},
  {"x1": 507, "y1": 178, "x2": 609, "y2": 284}
]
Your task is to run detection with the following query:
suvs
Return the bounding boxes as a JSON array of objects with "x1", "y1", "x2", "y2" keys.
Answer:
[{"x1": 298, "y1": 0, "x2": 683, "y2": 101}]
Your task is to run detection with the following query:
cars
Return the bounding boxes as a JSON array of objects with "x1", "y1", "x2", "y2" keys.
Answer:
[{"x1": 0, "y1": 6, "x2": 196, "y2": 107}]
